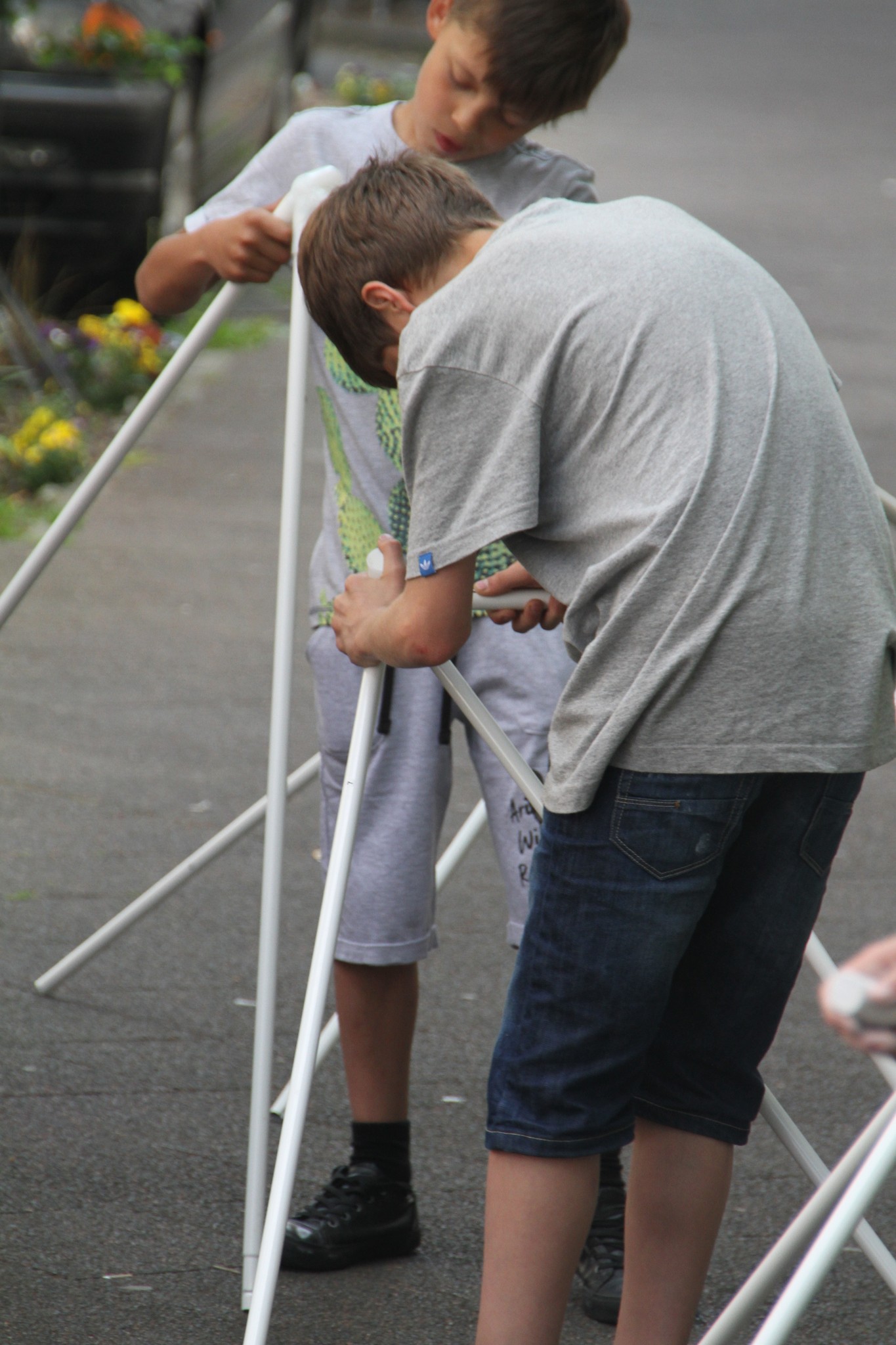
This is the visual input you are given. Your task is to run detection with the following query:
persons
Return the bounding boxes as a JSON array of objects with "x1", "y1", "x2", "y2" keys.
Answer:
[
  {"x1": 293, "y1": 144, "x2": 896, "y2": 1345},
  {"x1": 136, "y1": 0, "x2": 665, "y2": 1332},
  {"x1": 817, "y1": 923, "x2": 896, "y2": 1059}
]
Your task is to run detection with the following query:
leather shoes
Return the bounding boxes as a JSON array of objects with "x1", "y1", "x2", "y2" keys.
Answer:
[
  {"x1": 282, "y1": 1161, "x2": 423, "y2": 1269},
  {"x1": 574, "y1": 1185, "x2": 625, "y2": 1323}
]
